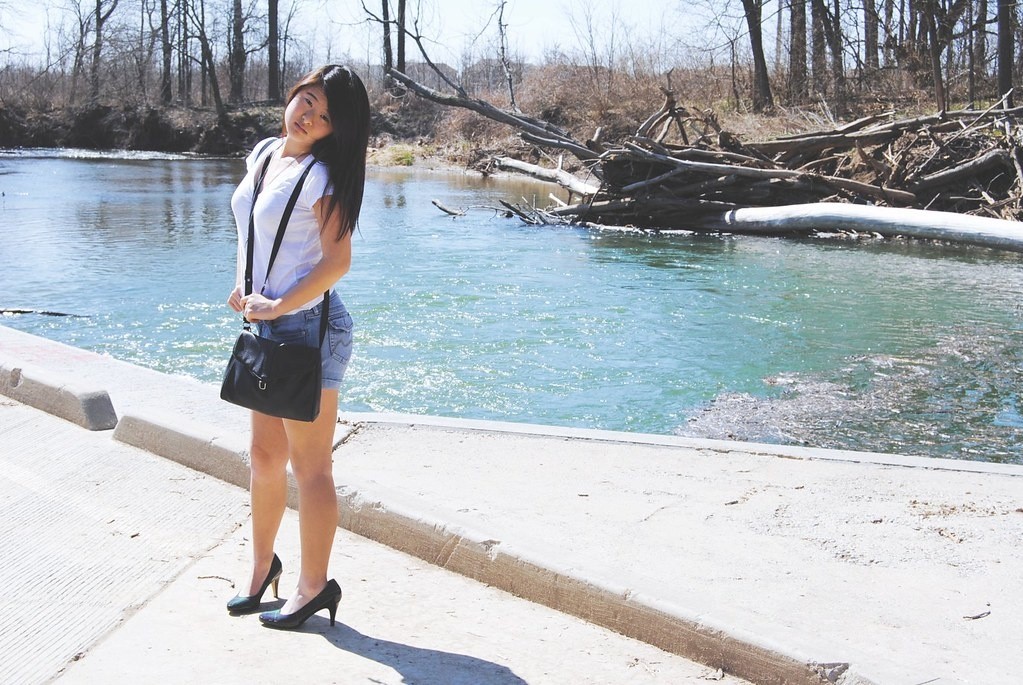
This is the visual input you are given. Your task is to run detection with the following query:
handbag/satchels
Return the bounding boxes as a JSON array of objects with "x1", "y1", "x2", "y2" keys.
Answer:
[{"x1": 220, "y1": 330, "x2": 321, "y2": 423}]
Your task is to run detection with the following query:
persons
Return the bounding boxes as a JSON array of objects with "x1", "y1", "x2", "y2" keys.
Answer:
[{"x1": 226, "y1": 64, "x2": 371, "y2": 630}]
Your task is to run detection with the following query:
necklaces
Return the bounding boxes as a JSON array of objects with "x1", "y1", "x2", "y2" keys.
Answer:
[{"x1": 244, "y1": 142, "x2": 304, "y2": 248}]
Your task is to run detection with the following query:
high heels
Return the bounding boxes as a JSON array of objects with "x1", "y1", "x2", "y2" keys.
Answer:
[
  {"x1": 227, "y1": 552, "x2": 283, "y2": 611},
  {"x1": 259, "y1": 578, "x2": 341, "y2": 629}
]
